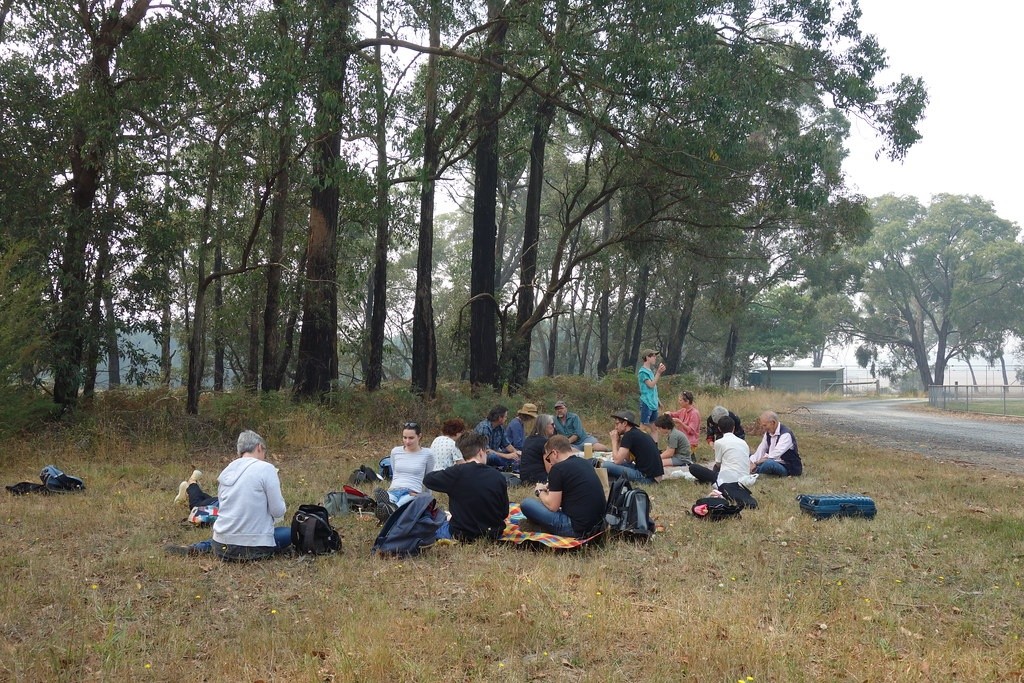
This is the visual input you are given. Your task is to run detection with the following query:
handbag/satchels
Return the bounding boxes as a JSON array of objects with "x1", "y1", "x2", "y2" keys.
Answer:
[{"x1": 717, "y1": 481, "x2": 758, "y2": 509}]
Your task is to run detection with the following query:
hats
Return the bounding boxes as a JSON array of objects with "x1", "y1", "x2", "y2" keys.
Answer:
[
  {"x1": 641, "y1": 348, "x2": 659, "y2": 357},
  {"x1": 555, "y1": 400, "x2": 567, "y2": 408},
  {"x1": 610, "y1": 410, "x2": 641, "y2": 426},
  {"x1": 517, "y1": 403, "x2": 538, "y2": 417}
]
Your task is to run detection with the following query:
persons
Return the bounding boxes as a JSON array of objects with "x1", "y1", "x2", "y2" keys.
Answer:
[
  {"x1": 375, "y1": 423, "x2": 434, "y2": 523},
  {"x1": 663, "y1": 390, "x2": 701, "y2": 452},
  {"x1": 173, "y1": 470, "x2": 220, "y2": 526},
  {"x1": 553, "y1": 401, "x2": 613, "y2": 452},
  {"x1": 638, "y1": 349, "x2": 666, "y2": 443},
  {"x1": 654, "y1": 413, "x2": 693, "y2": 467},
  {"x1": 423, "y1": 430, "x2": 509, "y2": 544},
  {"x1": 689, "y1": 416, "x2": 751, "y2": 487},
  {"x1": 750, "y1": 410, "x2": 803, "y2": 477},
  {"x1": 505, "y1": 403, "x2": 539, "y2": 450},
  {"x1": 165, "y1": 429, "x2": 291, "y2": 562},
  {"x1": 600, "y1": 410, "x2": 664, "y2": 486},
  {"x1": 520, "y1": 413, "x2": 556, "y2": 487},
  {"x1": 706, "y1": 406, "x2": 746, "y2": 449},
  {"x1": 519, "y1": 434, "x2": 607, "y2": 537},
  {"x1": 473, "y1": 404, "x2": 519, "y2": 466},
  {"x1": 430, "y1": 417, "x2": 466, "y2": 471}
]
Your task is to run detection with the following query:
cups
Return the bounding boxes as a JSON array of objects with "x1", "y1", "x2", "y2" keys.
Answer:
[{"x1": 584, "y1": 442, "x2": 592, "y2": 459}]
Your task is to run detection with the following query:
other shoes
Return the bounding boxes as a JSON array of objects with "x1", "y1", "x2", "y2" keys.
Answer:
[
  {"x1": 164, "y1": 544, "x2": 194, "y2": 558},
  {"x1": 174, "y1": 481, "x2": 189, "y2": 504},
  {"x1": 377, "y1": 502, "x2": 390, "y2": 525},
  {"x1": 519, "y1": 519, "x2": 546, "y2": 532},
  {"x1": 375, "y1": 488, "x2": 398, "y2": 514},
  {"x1": 188, "y1": 470, "x2": 203, "y2": 484}
]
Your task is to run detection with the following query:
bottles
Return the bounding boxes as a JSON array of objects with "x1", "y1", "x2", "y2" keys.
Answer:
[{"x1": 660, "y1": 363, "x2": 666, "y2": 370}]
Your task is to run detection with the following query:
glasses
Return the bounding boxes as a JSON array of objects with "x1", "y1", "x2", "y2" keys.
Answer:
[
  {"x1": 683, "y1": 390, "x2": 691, "y2": 402},
  {"x1": 545, "y1": 450, "x2": 559, "y2": 463},
  {"x1": 404, "y1": 422, "x2": 421, "y2": 430},
  {"x1": 528, "y1": 409, "x2": 537, "y2": 414},
  {"x1": 614, "y1": 421, "x2": 622, "y2": 426},
  {"x1": 547, "y1": 423, "x2": 554, "y2": 426}
]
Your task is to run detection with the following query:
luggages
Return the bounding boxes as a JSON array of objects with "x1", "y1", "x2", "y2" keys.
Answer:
[{"x1": 796, "y1": 493, "x2": 878, "y2": 520}]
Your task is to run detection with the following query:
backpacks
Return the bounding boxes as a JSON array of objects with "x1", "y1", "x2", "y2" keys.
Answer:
[
  {"x1": 371, "y1": 496, "x2": 447, "y2": 560},
  {"x1": 606, "y1": 472, "x2": 655, "y2": 544},
  {"x1": 691, "y1": 498, "x2": 742, "y2": 522},
  {"x1": 290, "y1": 503, "x2": 341, "y2": 555}
]
